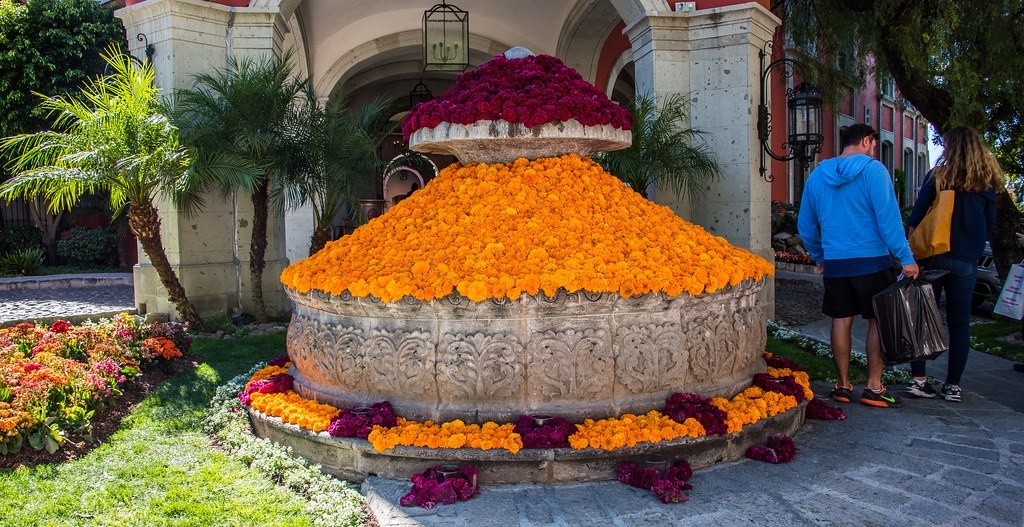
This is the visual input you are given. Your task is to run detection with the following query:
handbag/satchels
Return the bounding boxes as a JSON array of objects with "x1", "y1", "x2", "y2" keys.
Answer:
[
  {"x1": 993, "y1": 259, "x2": 1024, "y2": 320},
  {"x1": 908, "y1": 165, "x2": 955, "y2": 261},
  {"x1": 872, "y1": 276, "x2": 949, "y2": 367}
]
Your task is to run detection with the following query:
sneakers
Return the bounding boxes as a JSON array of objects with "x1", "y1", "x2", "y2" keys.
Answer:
[
  {"x1": 829, "y1": 382, "x2": 853, "y2": 403},
  {"x1": 903, "y1": 379, "x2": 937, "y2": 397},
  {"x1": 939, "y1": 384, "x2": 962, "y2": 401},
  {"x1": 860, "y1": 385, "x2": 903, "y2": 407}
]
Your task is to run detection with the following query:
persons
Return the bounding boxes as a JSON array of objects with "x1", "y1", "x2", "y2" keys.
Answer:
[
  {"x1": 1013, "y1": 232, "x2": 1024, "y2": 374},
  {"x1": 798, "y1": 123, "x2": 919, "y2": 407},
  {"x1": 905, "y1": 126, "x2": 1005, "y2": 402}
]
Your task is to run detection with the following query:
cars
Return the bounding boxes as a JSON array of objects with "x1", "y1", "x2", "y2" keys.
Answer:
[{"x1": 974, "y1": 241, "x2": 1007, "y2": 307}]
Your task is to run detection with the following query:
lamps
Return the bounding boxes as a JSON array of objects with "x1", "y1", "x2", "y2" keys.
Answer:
[
  {"x1": 421, "y1": 0, "x2": 470, "y2": 74},
  {"x1": 410, "y1": 78, "x2": 433, "y2": 110},
  {"x1": 757, "y1": 40, "x2": 824, "y2": 182}
]
